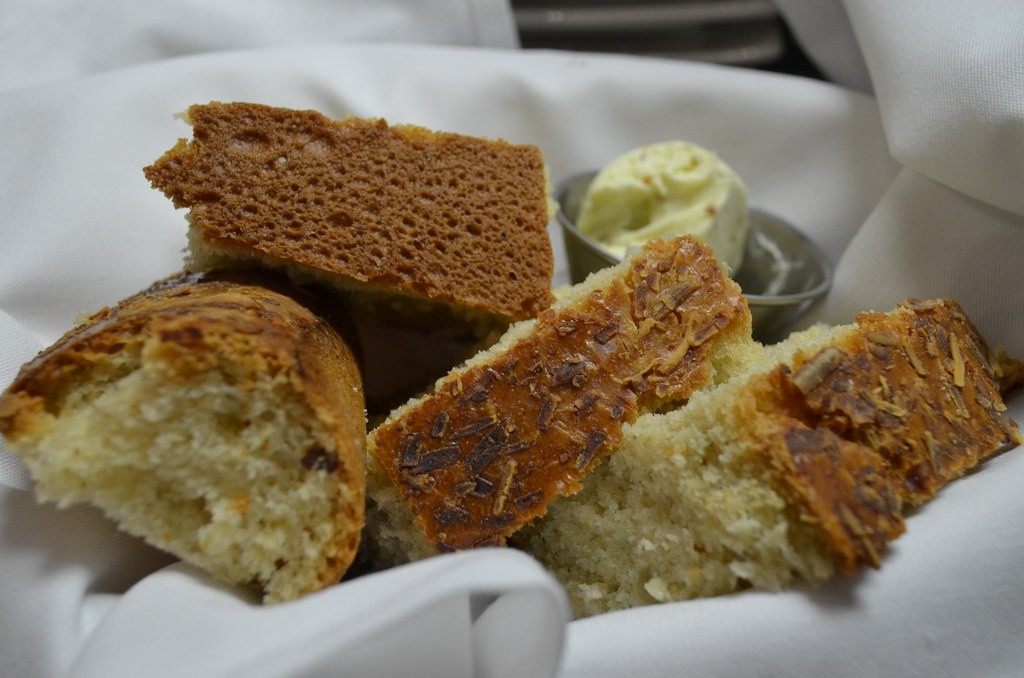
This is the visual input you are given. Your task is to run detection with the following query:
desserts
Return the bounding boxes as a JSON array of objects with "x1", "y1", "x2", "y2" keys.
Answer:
[{"x1": 574, "y1": 140, "x2": 752, "y2": 274}]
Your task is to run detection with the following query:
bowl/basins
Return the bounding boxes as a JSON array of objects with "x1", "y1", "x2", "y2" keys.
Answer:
[{"x1": 557, "y1": 169, "x2": 831, "y2": 349}]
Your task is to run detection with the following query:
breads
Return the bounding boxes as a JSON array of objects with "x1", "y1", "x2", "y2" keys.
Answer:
[
  {"x1": 508, "y1": 292, "x2": 1015, "y2": 610},
  {"x1": 366, "y1": 229, "x2": 748, "y2": 559},
  {"x1": 146, "y1": 102, "x2": 552, "y2": 399},
  {"x1": 0, "y1": 267, "x2": 369, "y2": 607}
]
